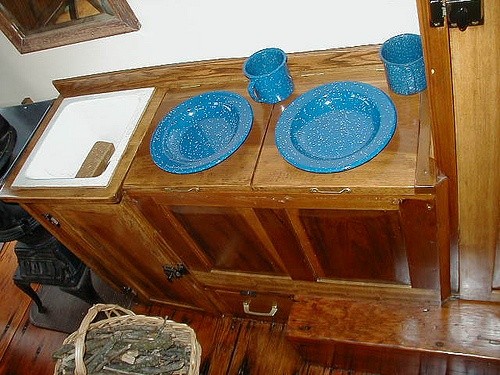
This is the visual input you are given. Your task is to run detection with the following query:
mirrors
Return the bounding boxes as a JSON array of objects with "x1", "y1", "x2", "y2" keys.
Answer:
[{"x1": 0, "y1": 0, "x2": 141, "y2": 54}]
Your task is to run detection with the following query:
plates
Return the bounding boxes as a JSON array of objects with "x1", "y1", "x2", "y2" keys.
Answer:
[
  {"x1": 149, "y1": 90, "x2": 254, "y2": 175},
  {"x1": 274, "y1": 80, "x2": 397, "y2": 175}
]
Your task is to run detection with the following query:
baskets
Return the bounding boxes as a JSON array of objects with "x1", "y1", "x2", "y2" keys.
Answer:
[{"x1": 54, "y1": 303, "x2": 202, "y2": 375}]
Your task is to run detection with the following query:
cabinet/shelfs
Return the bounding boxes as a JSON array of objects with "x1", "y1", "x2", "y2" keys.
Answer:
[{"x1": 19, "y1": 201, "x2": 445, "y2": 324}]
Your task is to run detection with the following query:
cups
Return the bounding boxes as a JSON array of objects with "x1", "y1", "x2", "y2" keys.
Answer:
[
  {"x1": 379, "y1": 32, "x2": 427, "y2": 96},
  {"x1": 242, "y1": 47, "x2": 295, "y2": 105}
]
